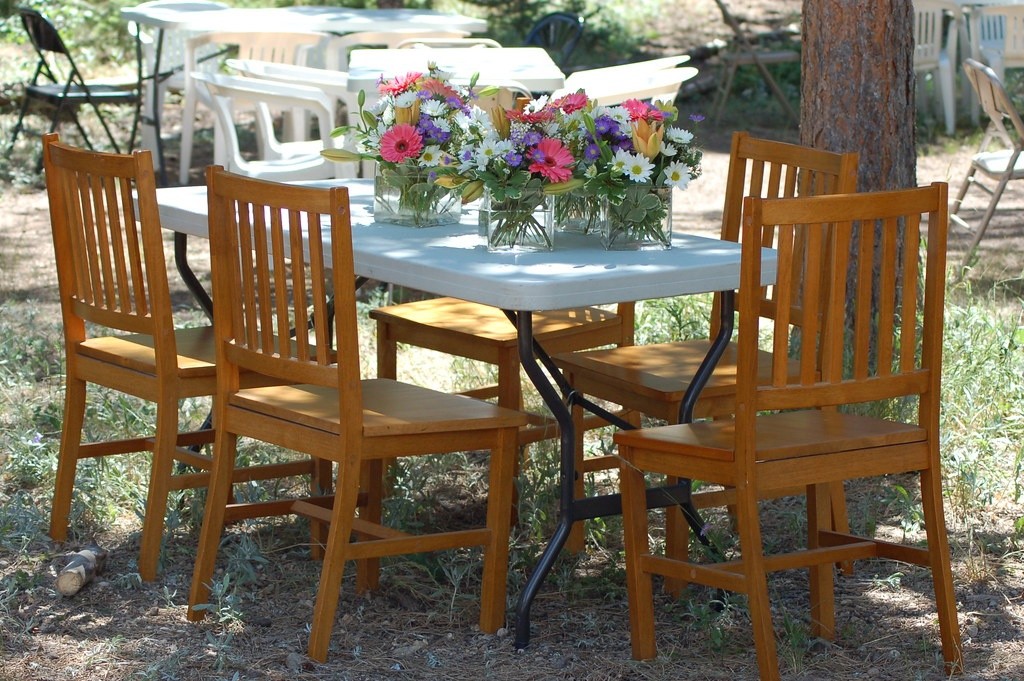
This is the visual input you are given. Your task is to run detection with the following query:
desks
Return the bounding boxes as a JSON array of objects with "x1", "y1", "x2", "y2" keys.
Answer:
[
  {"x1": 345, "y1": 45, "x2": 565, "y2": 123},
  {"x1": 178, "y1": 5, "x2": 491, "y2": 68},
  {"x1": 128, "y1": 177, "x2": 780, "y2": 650}
]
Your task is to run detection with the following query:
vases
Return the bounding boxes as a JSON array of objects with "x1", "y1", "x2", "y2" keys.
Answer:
[{"x1": 371, "y1": 160, "x2": 675, "y2": 256}]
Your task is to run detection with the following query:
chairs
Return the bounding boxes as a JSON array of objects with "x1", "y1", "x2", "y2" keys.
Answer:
[
  {"x1": 911, "y1": 0, "x2": 1024, "y2": 139},
  {"x1": 548, "y1": 130, "x2": 864, "y2": 603},
  {"x1": 947, "y1": 59, "x2": 1024, "y2": 281},
  {"x1": 367, "y1": 295, "x2": 644, "y2": 556},
  {"x1": 187, "y1": 165, "x2": 532, "y2": 665},
  {"x1": 42, "y1": 131, "x2": 335, "y2": 583},
  {"x1": 613, "y1": 180, "x2": 965, "y2": 680},
  {"x1": 2, "y1": 7, "x2": 142, "y2": 177},
  {"x1": 124, "y1": 0, "x2": 699, "y2": 187},
  {"x1": 708, "y1": 0, "x2": 801, "y2": 131}
]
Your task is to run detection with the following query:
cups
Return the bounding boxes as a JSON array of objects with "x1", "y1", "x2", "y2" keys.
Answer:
[{"x1": 54, "y1": 548, "x2": 106, "y2": 597}]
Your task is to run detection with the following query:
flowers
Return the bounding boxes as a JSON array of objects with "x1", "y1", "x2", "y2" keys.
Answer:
[{"x1": 314, "y1": 58, "x2": 706, "y2": 252}]
